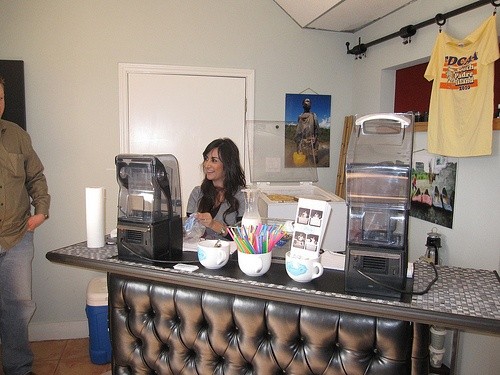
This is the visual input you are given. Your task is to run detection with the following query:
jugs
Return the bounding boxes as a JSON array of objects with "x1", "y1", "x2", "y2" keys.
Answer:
[{"x1": 241, "y1": 189, "x2": 262, "y2": 235}]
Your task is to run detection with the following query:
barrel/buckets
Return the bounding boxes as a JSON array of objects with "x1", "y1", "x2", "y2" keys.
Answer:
[{"x1": 85, "y1": 275, "x2": 111, "y2": 364}]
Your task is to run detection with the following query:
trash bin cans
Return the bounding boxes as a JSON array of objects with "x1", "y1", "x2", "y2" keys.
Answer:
[{"x1": 85, "y1": 276, "x2": 110, "y2": 365}]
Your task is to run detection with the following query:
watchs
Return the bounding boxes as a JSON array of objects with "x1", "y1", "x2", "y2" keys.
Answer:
[{"x1": 217, "y1": 224, "x2": 227, "y2": 235}]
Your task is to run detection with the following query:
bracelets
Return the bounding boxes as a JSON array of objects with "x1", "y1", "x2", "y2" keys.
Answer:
[{"x1": 43, "y1": 213, "x2": 48, "y2": 218}]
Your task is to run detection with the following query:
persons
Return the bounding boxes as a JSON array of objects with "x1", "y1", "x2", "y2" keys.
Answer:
[
  {"x1": 294, "y1": 98, "x2": 319, "y2": 163},
  {"x1": 186, "y1": 138, "x2": 248, "y2": 241},
  {"x1": 0, "y1": 78, "x2": 51, "y2": 375}
]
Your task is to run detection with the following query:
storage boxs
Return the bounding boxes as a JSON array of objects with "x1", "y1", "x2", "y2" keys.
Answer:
[{"x1": 85, "y1": 277, "x2": 111, "y2": 365}]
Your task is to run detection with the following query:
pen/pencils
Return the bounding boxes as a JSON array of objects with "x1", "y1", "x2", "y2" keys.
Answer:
[{"x1": 214, "y1": 239, "x2": 220, "y2": 247}]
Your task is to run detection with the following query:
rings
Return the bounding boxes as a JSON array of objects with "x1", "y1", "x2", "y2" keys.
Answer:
[{"x1": 204, "y1": 219, "x2": 207, "y2": 222}]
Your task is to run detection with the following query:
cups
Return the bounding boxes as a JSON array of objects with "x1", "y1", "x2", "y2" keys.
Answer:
[
  {"x1": 285, "y1": 251, "x2": 324, "y2": 283},
  {"x1": 197, "y1": 240, "x2": 230, "y2": 270},
  {"x1": 237, "y1": 249, "x2": 272, "y2": 277}
]
